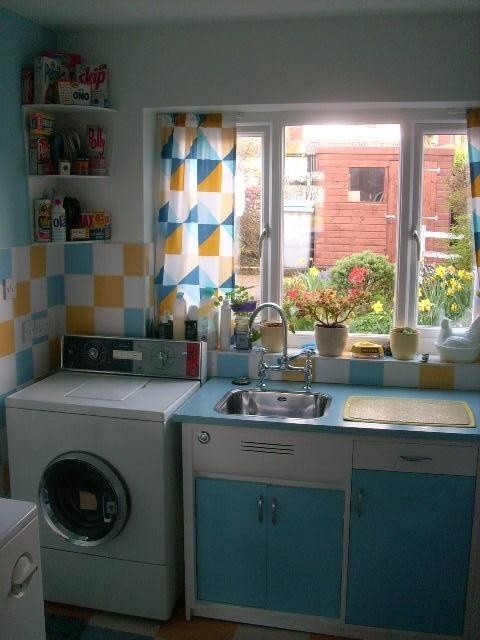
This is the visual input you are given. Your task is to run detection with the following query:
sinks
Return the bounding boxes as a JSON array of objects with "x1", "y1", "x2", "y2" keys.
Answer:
[{"x1": 213, "y1": 387, "x2": 333, "y2": 422}]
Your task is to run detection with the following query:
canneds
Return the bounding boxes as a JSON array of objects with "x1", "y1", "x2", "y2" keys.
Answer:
[{"x1": 76, "y1": 157, "x2": 88, "y2": 175}]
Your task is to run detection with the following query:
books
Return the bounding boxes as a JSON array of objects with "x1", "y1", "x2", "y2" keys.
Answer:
[{"x1": 28, "y1": 112, "x2": 56, "y2": 176}]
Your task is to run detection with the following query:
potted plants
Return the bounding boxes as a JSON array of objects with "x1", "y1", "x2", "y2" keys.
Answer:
[{"x1": 214, "y1": 284, "x2": 257, "y2": 312}]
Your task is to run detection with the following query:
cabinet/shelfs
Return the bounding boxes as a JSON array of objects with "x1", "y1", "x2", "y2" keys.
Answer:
[
  {"x1": 23, "y1": 102, "x2": 119, "y2": 246},
  {"x1": 344, "y1": 425, "x2": 480, "y2": 639},
  {"x1": 174, "y1": 413, "x2": 351, "y2": 638}
]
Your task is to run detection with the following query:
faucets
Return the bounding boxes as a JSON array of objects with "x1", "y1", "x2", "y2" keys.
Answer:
[{"x1": 248, "y1": 303, "x2": 314, "y2": 391}]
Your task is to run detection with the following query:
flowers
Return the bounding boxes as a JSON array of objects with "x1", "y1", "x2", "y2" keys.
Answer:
[
  {"x1": 285, "y1": 267, "x2": 370, "y2": 335},
  {"x1": 372, "y1": 262, "x2": 474, "y2": 322}
]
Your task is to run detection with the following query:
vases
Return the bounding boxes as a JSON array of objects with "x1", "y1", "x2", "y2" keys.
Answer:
[
  {"x1": 313, "y1": 323, "x2": 348, "y2": 357},
  {"x1": 391, "y1": 326, "x2": 421, "y2": 361}
]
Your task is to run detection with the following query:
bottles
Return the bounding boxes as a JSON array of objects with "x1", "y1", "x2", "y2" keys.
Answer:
[{"x1": 173, "y1": 292, "x2": 186, "y2": 341}]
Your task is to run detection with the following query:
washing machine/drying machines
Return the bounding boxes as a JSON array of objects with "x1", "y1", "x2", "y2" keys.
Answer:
[{"x1": 1, "y1": 331, "x2": 209, "y2": 622}]
